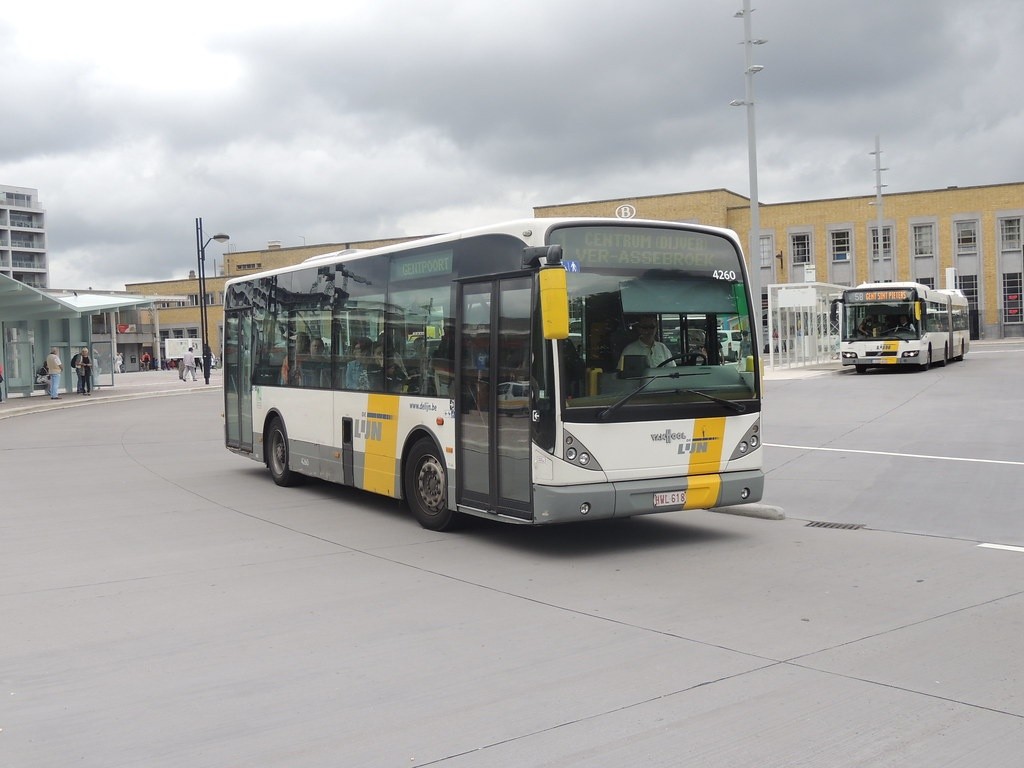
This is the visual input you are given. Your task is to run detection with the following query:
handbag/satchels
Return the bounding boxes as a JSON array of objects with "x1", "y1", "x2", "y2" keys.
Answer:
[
  {"x1": 37, "y1": 367, "x2": 49, "y2": 383},
  {"x1": 76, "y1": 355, "x2": 82, "y2": 368},
  {"x1": 0, "y1": 374, "x2": 3, "y2": 383}
]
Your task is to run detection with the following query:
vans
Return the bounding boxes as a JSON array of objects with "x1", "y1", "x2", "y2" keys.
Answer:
[{"x1": 717, "y1": 330, "x2": 744, "y2": 362}]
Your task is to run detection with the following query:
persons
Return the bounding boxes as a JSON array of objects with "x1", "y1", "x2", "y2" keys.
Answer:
[
  {"x1": 281, "y1": 332, "x2": 325, "y2": 385},
  {"x1": 738, "y1": 331, "x2": 752, "y2": 362},
  {"x1": 0, "y1": 362, "x2": 6, "y2": 404},
  {"x1": 114, "y1": 353, "x2": 126, "y2": 375},
  {"x1": 894, "y1": 315, "x2": 916, "y2": 333},
  {"x1": 618, "y1": 314, "x2": 677, "y2": 371},
  {"x1": 702, "y1": 341, "x2": 725, "y2": 366},
  {"x1": 859, "y1": 311, "x2": 882, "y2": 335},
  {"x1": 140, "y1": 352, "x2": 165, "y2": 372},
  {"x1": 39, "y1": 347, "x2": 64, "y2": 399},
  {"x1": 346, "y1": 333, "x2": 426, "y2": 391},
  {"x1": 75, "y1": 347, "x2": 92, "y2": 395},
  {"x1": 182, "y1": 347, "x2": 198, "y2": 382}
]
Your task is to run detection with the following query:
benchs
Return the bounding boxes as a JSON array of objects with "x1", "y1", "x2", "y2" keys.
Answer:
[{"x1": 33, "y1": 383, "x2": 49, "y2": 395}]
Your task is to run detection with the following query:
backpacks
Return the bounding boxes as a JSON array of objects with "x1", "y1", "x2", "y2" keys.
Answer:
[{"x1": 71, "y1": 354, "x2": 78, "y2": 368}]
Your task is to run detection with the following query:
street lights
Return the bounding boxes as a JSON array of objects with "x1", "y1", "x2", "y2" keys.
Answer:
[
  {"x1": 195, "y1": 217, "x2": 230, "y2": 350},
  {"x1": 868, "y1": 128, "x2": 888, "y2": 323},
  {"x1": 729, "y1": 0, "x2": 769, "y2": 357}
]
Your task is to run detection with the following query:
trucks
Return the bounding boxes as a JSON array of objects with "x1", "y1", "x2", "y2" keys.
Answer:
[{"x1": 165, "y1": 338, "x2": 215, "y2": 367}]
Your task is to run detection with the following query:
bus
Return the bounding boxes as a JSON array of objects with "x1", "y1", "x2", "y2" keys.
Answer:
[
  {"x1": 497, "y1": 381, "x2": 530, "y2": 417},
  {"x1": 221, "y1": 217, "x2": 765, "y2": 532},
  {"x1": 830, "y1": 282, "x2": 969, "y2": 374}
]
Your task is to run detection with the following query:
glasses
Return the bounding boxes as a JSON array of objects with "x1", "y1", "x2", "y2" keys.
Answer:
[{"x1": 639, "y1": 324, "x2": 657, "y2": 329}]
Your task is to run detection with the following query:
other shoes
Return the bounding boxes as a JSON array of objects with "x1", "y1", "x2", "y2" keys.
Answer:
[
  {"x1": 193, "y1": 379, "x2": 198, "y2": 381},
  {"x1": 84, "y1": 392, "x2": 87, "y2": 395},
  {"x1": 88, "y1": 393, "x2": 91, "y2": 395},
  {"x1": 183, "y1": 379, "x2": 186, "y2": 382},
  {"x1": 0, "y1": 401, "x2": 6, "y2": 404},
  {"x1": 51, "y1": 397, "x2": 62, "y2": 399}
]
